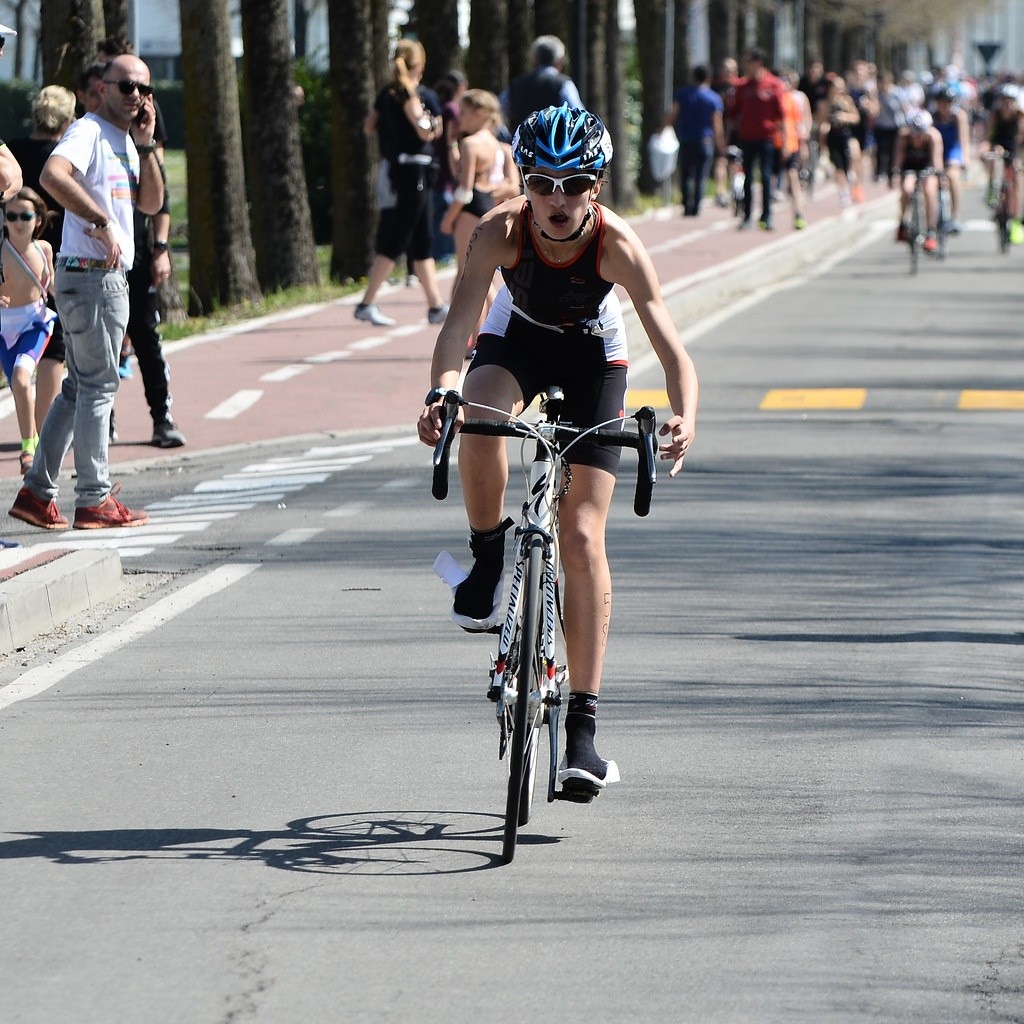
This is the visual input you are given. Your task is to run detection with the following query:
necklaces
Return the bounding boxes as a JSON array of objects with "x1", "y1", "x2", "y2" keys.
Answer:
[{"x1": 536, "y1": 232, "x2": 588, "y2": 264}]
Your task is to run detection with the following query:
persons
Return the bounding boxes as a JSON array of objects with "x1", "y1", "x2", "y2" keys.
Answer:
[
  {"x1": 663, "y1": 63, "x2": 729, "y2": 219},
  {"x1": 79, "y1": 61, "x2": 187, "y2": 448},
  {"x1": 0, "y1": 19, "x2": 22, "y2": 210},
  {"x1": 352, "y1": 37, "x2": 453, "y2": 329},
  {"x1": 92, "y1": 30, "x2": 165, "y2": 178},
  {"x1": 498, "y1": 34, "x2": 590, "y2": 137},
  {"x1": 716, "y1": 48, "x2": 1024, "y2": 255},
  {"x1": 434, "y1": 67, "x2": 524, "y2": 362},
  {"x1": 8, "y1": 54, "x2": 165, "y2": 530},
  {"x1": 418, "y1": 105, "x2": 699, "y2": 790},
  {"x1": 24, "y1": 78, "x2": 79, "y2": 437},
  {"x1": 0, "y1": 187, "x2": 55, "y2": 478}
]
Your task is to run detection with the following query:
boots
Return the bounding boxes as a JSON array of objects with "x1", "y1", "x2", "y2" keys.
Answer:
[
  {"x1": 432, "y1": 516, "x2": 515, "y2": 630},
  {"x1": 558, "y1": 691, "x2": 621, "y2": 788}
]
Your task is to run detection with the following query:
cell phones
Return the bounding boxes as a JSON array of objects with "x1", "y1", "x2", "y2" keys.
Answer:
[{"x1": 136, "y1": 103, "x2": 147, "y2": 128}]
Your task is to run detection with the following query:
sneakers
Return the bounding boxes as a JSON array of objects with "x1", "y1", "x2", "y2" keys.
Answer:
[
  {"x1": 72, "y1": 482, "x2": 148, "y2": 529},
  {"x1": 354, "y1": 303, "x2": 395, "y2": 326},
  {"x1": 108, "y1": 421, "x2": 118, "y2": 446},
  {"x1": 119, "y1": 355, "x2": 132, "y2": 378},
  {"x1": 428, "y1": 304, "x2": 449, "y2": 324},
  {"x1": 152, "y1": 422, "x2": 185, "y2": 447},
  {"x1": 9, "y1": 487, "x2": 68, "y2": 529}
]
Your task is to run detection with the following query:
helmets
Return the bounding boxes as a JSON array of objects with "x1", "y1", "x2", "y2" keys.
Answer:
[
  {"x1": 932, "y1": 84, "x2": 956, "y2": 101},
  {"x1": 511, "y1": 100, "x2": 613, "y2": 172},
  {"x1": 999, "y1": 84, "x2": 1020, "y2": 101},
  {"x1": 905, "y1": 105, "x2": 932, "y2": 129}
]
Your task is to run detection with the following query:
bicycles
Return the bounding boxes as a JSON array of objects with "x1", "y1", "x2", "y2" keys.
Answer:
[
  {"x1": 424, "y1": 382, "x2": 659, "y2": 862},
  {"x1": 981, "y1": 149, "x2": 1019, "y2": 255},
  {"x1": 887, "y1": 159, "x2": 954, "y2": 277}
]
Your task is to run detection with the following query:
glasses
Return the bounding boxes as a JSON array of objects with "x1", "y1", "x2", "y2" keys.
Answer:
[
  {"x1": 520, "y1": 166, "x2": 599, "y2": 196},
  {"x1": 5, "y1": 210, "x2": 37, "y2": 222},
  {"x1": 103, "y1": 80, "x2": 153, "y2": 96}
]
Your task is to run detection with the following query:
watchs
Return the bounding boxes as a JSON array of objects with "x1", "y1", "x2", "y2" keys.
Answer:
[
  {"x1": 153, "y1": 241, "x2": 168, "y2": 252},
  {"x1": 423, "y1": 387, "x2": 448, "y2": 407},
  {"x1": 87, "y1": 218, "x2": 116, "y2": 230},
  {"x1": 136, "y1": 138, "x2": 158, "y2": 154}
]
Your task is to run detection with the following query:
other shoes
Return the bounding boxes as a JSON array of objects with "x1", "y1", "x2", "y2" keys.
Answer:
[
  {"x1": 759, "y1": 220, "x2": 769, "y2": 230},
  {"x1": 1007, "y1": 218, "x2": 1024, "y2": 244},
  {"x1": 19, "y1": 450, "x2": 33, "y2": 474},
  {"x1": 794, "y1": 219, "x2": 807, "y2": 229},
  {"x1": 898, "y1": 221, "x2": 910, "y2": 240},
  {"x1": 949, "y1": 218, "x2": 960, "y2": 232},
  {"x1": 739, "y1": 223, "x2": 751, "y2": 230},
  {"x1": 923, "y1": 230, "x2": 937, "y2": 251}
]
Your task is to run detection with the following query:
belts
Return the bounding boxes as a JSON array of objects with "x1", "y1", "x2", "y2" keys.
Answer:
[{"x1": 55, "y1": 257, "x2": 124, "y2": 277}]
